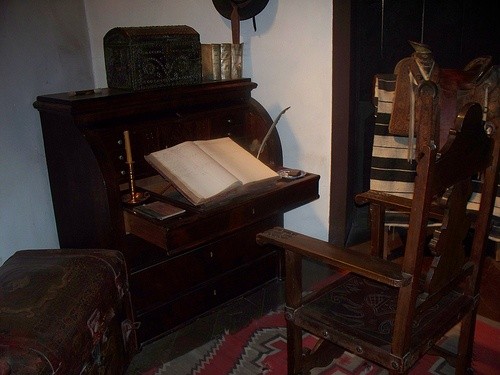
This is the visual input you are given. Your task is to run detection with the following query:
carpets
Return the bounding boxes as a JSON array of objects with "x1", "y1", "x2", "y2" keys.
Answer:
[{"x1": 134, "y1": 268, "x2": 500, "y2": 375}]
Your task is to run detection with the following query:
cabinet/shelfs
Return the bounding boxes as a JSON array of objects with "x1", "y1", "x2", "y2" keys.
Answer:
[{"x1": 33, "y1": 78, "x2": 320, "y2": 352}]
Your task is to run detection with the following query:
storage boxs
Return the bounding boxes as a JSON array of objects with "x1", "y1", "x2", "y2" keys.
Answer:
[
  {"x1": 0, "y1": 249, "x2": 137, "y2": 375},
  {"x1": 103, "y1": 25, "x2": 203, "y2": 94}
]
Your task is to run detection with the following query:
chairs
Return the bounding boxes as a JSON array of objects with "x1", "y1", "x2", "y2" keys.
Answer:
[{"x1": 257, "y1": 80, "x2": 500, "y2": 375}]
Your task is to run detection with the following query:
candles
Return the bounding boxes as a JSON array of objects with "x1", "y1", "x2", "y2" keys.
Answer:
[{"x1": 124, "y1": 130, "x2": 132, "y2": 162}]
